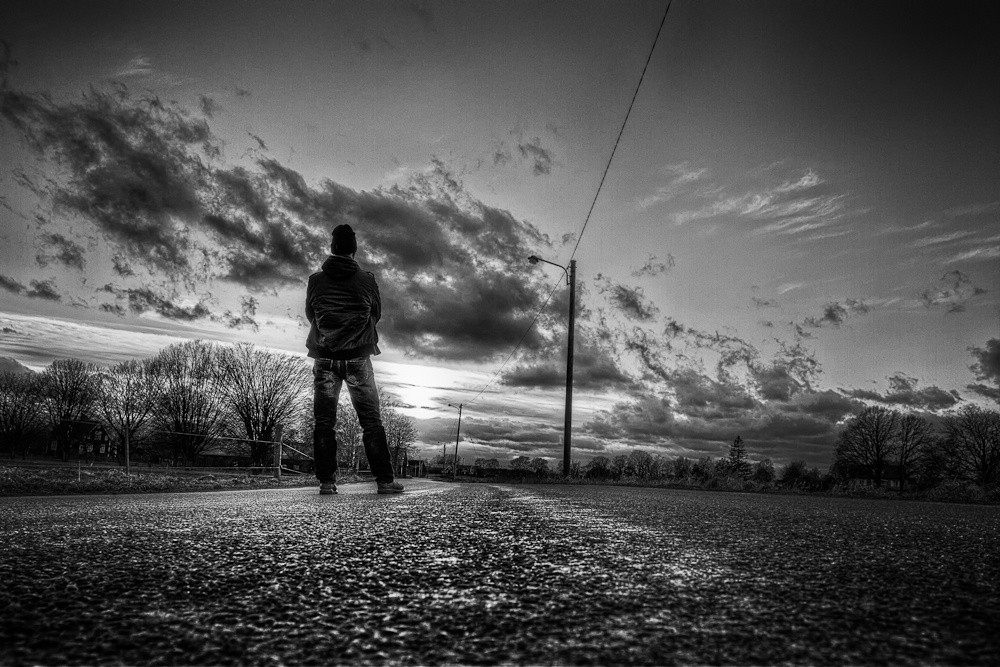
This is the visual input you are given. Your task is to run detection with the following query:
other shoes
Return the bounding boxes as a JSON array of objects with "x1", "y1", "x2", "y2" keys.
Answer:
[
  {"x1": 377, "y1": 481, "x2": 405, "y2": 493},
  {"x1": 319, "y1": 483, "x2": 338, "y2": 494}
]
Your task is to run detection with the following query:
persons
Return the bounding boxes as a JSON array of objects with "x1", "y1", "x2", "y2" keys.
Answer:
[{"x1": 305, "y1": 224, "x2": 405, "y2": 495}]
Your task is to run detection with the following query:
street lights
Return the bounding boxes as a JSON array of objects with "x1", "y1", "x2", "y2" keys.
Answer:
[
  {"x1": 448, "y1": 403, "x2": 461, "y2": 481},
  {"x1": 527, "y1": 254, "x2": 576, "y2": 477}
]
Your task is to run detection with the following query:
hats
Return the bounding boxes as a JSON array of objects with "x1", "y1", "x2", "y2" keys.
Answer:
[{"x1": 330, "y1": 225, "x2": 358, "y2": 257}]
